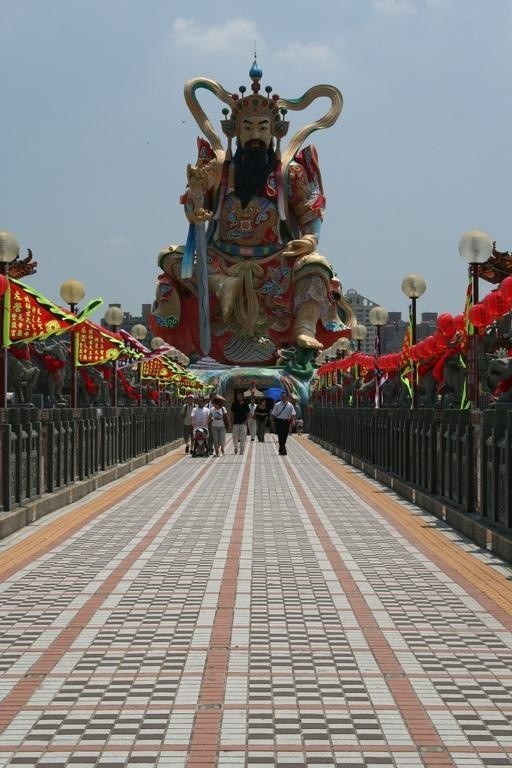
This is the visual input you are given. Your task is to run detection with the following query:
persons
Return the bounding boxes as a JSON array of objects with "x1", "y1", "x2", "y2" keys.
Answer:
[
  {"x1": 153, "y1": 60, "x2": 357, "y2": 350},
  {"x1": 182, "y1": 389, "x2": 305, "y2": 457}
]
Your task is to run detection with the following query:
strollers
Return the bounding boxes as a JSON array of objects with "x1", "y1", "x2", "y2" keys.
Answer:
[{"x1": 192, "y1": 425, "x2": 209, "y2": 457}]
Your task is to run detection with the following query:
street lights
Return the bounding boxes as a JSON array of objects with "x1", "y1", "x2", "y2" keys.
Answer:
[
  {"x1": 151, "y1": 336, "x2": 190, "y2": 407},
  {"x1": 401, "y1": 272, "x2": 427, "y2": 406},
  {"x1": 369, "y1": 306, "x2": 391, "y2": 406},
  {"x1": 60, "y1": 277, "x2": 85, "y2": 406},
  {"x1": 351, "y1": 324, "x2": 368, "y2": 406},
  {"x1": 459, "y1": 229, "x2": 494, "y2": 410},
  {"x1": 130, "y1": 323, "x2": 147, "y2": 406},
  {"x1": 104, "y1": 306, "x2": 124, "y2": 406},
  {"x1": 0, "y1": 230, "x2": 19, "y2": 407},
  {"x1": 315, "y1": 336, "x2": 350, "y2": 407}
]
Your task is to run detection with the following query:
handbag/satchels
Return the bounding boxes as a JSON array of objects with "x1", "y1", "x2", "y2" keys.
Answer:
[{"x1": 224, "y1": 413, "x2": 231, "y2": 430}]
